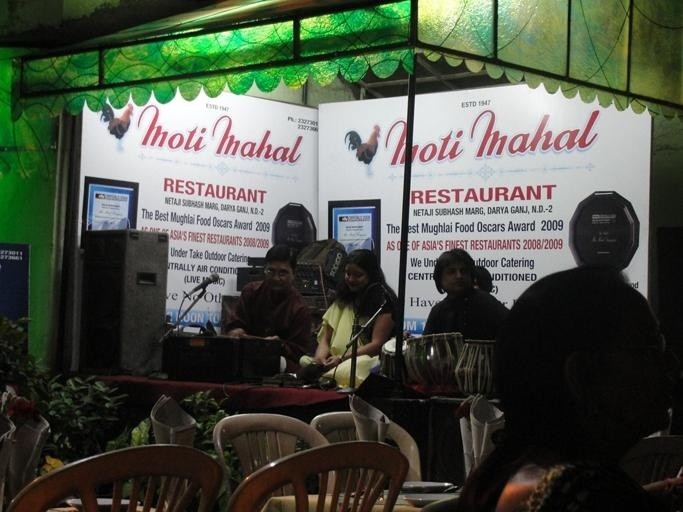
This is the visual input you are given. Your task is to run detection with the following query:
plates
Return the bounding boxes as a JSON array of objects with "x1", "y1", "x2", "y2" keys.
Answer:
[
  {"x1": 400, "y1": 481, "x2": 454, "y2": 493},
  {"x1": 398, "y1": 494, "x2": 459, "y2": 508},
  {"x1": 66, "y1": 498, "x2": 141, "y2": 510}
]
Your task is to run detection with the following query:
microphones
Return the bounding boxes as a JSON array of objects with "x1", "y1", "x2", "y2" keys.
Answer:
[
  {"x1": 373, "y1": 277, "x2": 400, "y2": 313},
  {"x1": 184, "y1": 273, "x2": 222, "y2": 297}
]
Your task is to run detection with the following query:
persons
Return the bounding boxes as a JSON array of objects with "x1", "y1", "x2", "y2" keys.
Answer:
[
  {"x1": 226, "y1": 246, "x2": 314, "y2": 349},
  {"x1": 472, "y1": 267, "x2": 492, "y2": 295},
  {"x1": 304, "y1": 249, "x2": 398, "y2": 383},
  {"x1": 422, "y1": 248, "x2": 512, "y2": 351}
]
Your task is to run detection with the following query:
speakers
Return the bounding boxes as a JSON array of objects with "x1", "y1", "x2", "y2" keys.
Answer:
[{"x1": 78, "y1": 227, "x2": 169, "y2": 375}]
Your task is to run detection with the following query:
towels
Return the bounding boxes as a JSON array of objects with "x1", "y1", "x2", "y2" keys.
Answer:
[
  {"x1": 150, "y1": 394, "x2": 196, "y2": 511},
  {"x1": 459, "y1": 396, "x2": 472, "y2": 482},
  {"x1": 0, "y1": 385, "x2": 50, "y2": 508},
  {"x1": 471, "y1": 392, "x2": 506, "y2": 470},
  {"x1": 349, "y1": 395, "x2": 389, "y2": 500}
]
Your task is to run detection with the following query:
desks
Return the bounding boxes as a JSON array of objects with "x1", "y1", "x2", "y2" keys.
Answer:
[{"x1": 103, "y1": 373, "x2": 505, "y2": 483}]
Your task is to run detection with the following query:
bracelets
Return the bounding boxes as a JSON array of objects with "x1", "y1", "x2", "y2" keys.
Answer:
[{"x1": 340, "y1": 356, "x2": 345, "y2": 363}]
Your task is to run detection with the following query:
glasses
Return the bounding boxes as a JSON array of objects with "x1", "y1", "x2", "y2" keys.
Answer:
[{"x1": 265, "y1": 270, "x2": 292, "y2": 276}]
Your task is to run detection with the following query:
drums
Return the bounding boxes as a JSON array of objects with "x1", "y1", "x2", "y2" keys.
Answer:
[
  {"x1": 380, "y1": 336, "x2": 408, "y2": 380},
  {"x1": 407, "y1": 333, "x2": 464, "y2": 385},
  {"x1": 455, "y1": 340, "x2": 494, "y2": 396}
]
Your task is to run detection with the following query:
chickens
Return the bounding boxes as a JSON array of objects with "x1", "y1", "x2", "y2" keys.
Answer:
[
  {"x1": 100, "y1": 103, "x2": 132, "y2": 140},
  {"x1": 344, "y1": 125, "x2": 380, "y2": 165}
]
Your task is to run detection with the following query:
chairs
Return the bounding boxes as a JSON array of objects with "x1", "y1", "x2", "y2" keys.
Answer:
[
  {"x1": 227, "y1": 439, "x2": 409, "y2": 512},
  {"x1": 7, "y1": 444, "x2": 222, "y2": 511},
  {"x1": 311, "y1": 413, "x2": 421, "y2": 485},
  {"x1": 214, "y1": 413, "x2": 338, "y2": 502},
  {"x1": 616, "y1": 437, "x2": 682, "y2": 493}
]
